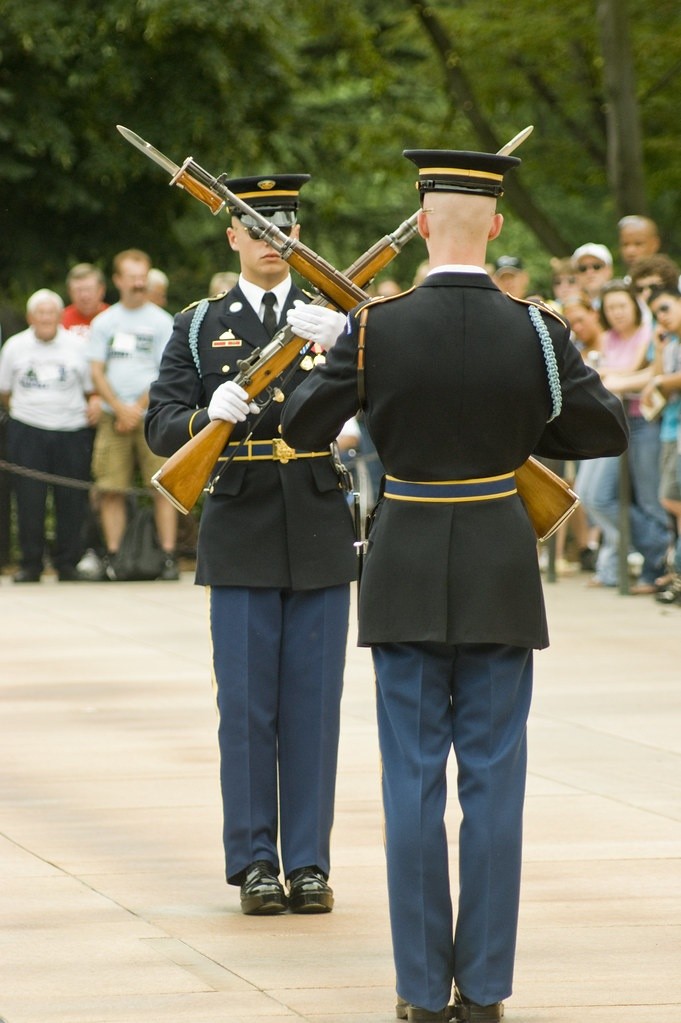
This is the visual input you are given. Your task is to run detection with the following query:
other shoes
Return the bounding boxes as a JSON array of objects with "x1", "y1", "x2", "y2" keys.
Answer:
[
  {"x1": 15, "y1": 567, "x2": 40, "y2": 582},
  {"x1": 59, "y1": 566, "x2": 78, "y2": 580},
  {"x1": 162, "y1": 551, "x2": 178, "y2": 580}
]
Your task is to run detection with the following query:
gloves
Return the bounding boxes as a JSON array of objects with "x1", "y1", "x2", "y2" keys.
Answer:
[
  {"x1": 207, "y1": 380, "x2": 260, "y2": 423},
  {"x1": 285, "y1": 299, "x2": 348, "y2": 352}
]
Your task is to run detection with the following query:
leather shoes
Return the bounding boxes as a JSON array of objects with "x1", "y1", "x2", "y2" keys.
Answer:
[
  {"x1": 395, "y1": 984, "x2": 505, "y2": 1023},
  {"x1": 240, "y1": 860, "x2": 288, "y2": 913},
  {"x1": 285, "y1": 867, "x2": 333, "y2": 913}
]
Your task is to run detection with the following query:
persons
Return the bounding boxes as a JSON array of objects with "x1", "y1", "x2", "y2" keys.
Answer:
[
  {"x1": 0, "y1": 212, "x2": 681, "y2": 605},
  {"x1": 142, "y1": 172, "x2": 362, "y2": 915},
  {"x1": 274, "y1": 147, "x2": 632, "y2": 1023}
]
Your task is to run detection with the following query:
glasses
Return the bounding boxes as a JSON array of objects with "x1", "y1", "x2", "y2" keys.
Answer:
[
  {"x1": 636, "y1": 284, "x2": 656, "y2": 292},
  {"x1": 575, "y1": 263, "x2": 602, "y2": 271},
  {"x1": 240, "y1": 227, "x2": 293, "y2": 240}
]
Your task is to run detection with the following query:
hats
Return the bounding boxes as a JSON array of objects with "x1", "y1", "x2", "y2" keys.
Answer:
[
  {"x1": 571, "y1": 242, "x2": 612, "y2": 265},
  {"x1": 402, "y1": 150, "x2": 521, "y2": 197},
  {"x1": 223, "y1": 173, "x2": 312, "y2": 216},
  {"x1": 492, "y1": 255, "x2": 520, "y2": 280}
]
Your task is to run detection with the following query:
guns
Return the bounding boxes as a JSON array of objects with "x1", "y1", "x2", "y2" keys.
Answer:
[
  {"x1": 148, "y1": 126, "x2": 538, "y2": 517},
  {"x1": 113, "y1": 119, "x2": 578, "y2": 544}
]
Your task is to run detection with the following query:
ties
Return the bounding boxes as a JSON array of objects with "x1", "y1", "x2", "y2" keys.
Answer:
[{"x1": 263, "y1": 292, "x2": 277, "y2": 340}]
